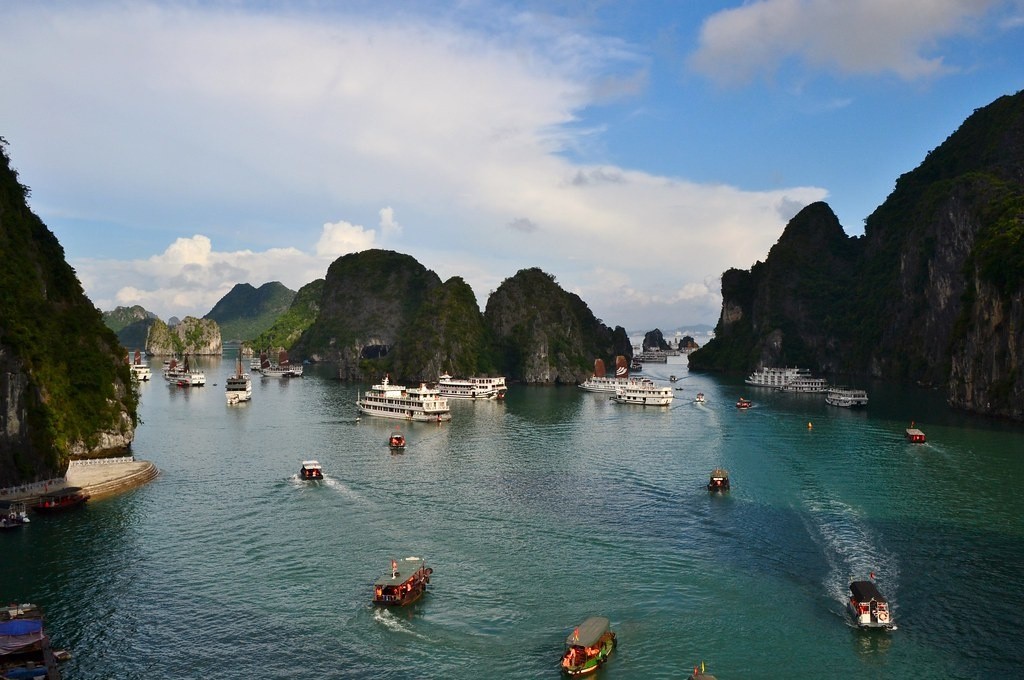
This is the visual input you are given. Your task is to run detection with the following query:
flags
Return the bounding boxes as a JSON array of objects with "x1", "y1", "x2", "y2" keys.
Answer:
[
  {"x1": 574, "y1": 628, "x2": 579, "y2": 641},
  {"x1": 701, "y1": 661, "x2": 705, "y2": 674},
  {"x1": 393, "y1": 561, "x2": 397, "y2": 572},
  {"x1": 694, "y1": 666, "x2": 698, "y2": 676}
]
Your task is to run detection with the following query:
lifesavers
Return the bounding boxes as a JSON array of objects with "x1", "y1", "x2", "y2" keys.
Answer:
[
  {"x1": 879, "y1": 613, "x2": 887, "y2": 621},
  {"x1": 392, "y1": 571, "x2": 400, "y2": 576},
  {"x1": 426, "y1": 568, "x2": 433, "y2": 574}
]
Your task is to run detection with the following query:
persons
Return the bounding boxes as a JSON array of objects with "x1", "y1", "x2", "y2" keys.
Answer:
[
  {"x1": 389, "y1": 436, "x2": 405, "y2": 446},
  {"x1": 416, "y1": 568, "x2": 433, "y2": 576},
  {"x1": 304, "y1": 468, "x2": 317, "y2": 477},
  {"x1": 567, "y1": 647, "x2": 576, "y2": 666},
  {"x1": 376, "y1": 586, "x2": 383, "y2": 600},
  {"x1": 414, "y1": 574, "x2": 430, "y2": 585},
  {"x1": 44, "y1": 494, "x2": 79, "y2": 507}
]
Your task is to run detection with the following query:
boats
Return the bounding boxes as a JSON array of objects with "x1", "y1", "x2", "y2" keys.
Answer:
[
  {"x1": 0, "y1": 486, "x2": 91, "y2": 529},
  {"x1": 743, "y1": 367, "x2": 869, "y2": 406},
  {"x1": 162, "y1": 352, "x2": 206, "y2": 386},
  {"x1": 706, "y1": 467, "x2": 730, "y2": 491},
  {"x1": 388, "y1": 431, "x2": 406, "y2": 450},
  {"x1": 369, "y1": 554, "x2": 433, "y2": 607},
  {"x1": 558, "y1": 616, "x2": 618, "y2": 679},
  {"x1": 695, "y1": 393, "x2": 705, "y2": 402},
  {"x1": 736, "y1": 398, "x2": 752, "y2": 408},
  {"x1": 356, "y1": 373, "x2": 452, "y2": 422},
  {"x1": 0, "y1": 596, "x2": 62, "y2": 680},
  {"x1": 846, "y1": 580, "x2": 894, "y2": 629},
  {"x1": 250, "y1": 358, "x2": 304, "y2": 377},
  {"x1": 577, "y1": 375, "x2": 675, "y2": 405},
  {"x1": 433, "y1": 370, "x2": 508, "y2": 400},
  {"x1": 129, "y1": 362, "x2": 152, "y2": 380},
  {"x1": 225, "y1": 346, "x2": 252, "y2": 404},
  {"x1": 631, "y1": 347, "x2": 696, "y2": 372},
  {"x1": 903, "y1": 428, "x2": 926, "y2": 443},
  {"x1": 300, "y1": 460, "x2": 324, "y2": 480},
  {"x1": 687, "y1": 673, "x2": 717, "y2": 680}
]
